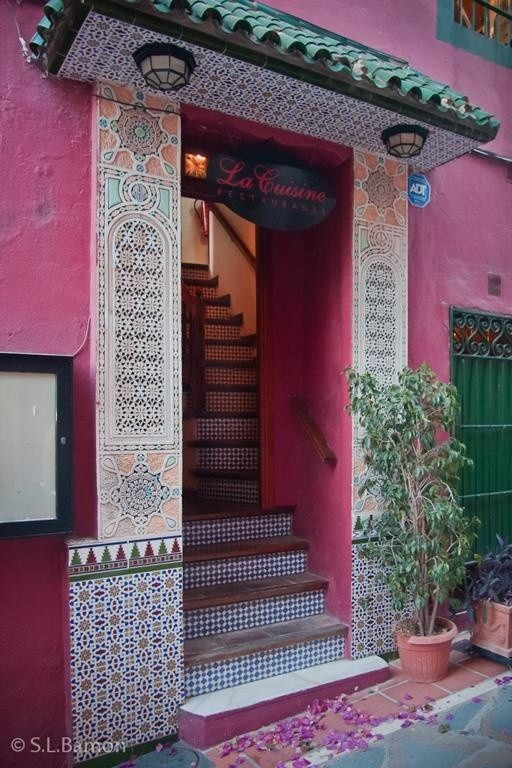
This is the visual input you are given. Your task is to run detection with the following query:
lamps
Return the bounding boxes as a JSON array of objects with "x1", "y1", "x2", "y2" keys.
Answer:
[
  {"x1": 381, "y1": 126, "x2": 429, "y2": 160},
  {"x1": 132, "y1": 42, "x2": 199, "y2": 92}
]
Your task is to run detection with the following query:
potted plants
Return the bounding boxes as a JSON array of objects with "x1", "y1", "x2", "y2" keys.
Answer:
[
  {"x1": 445, "y1": 535, "x2": 512, "y2": 667},
  {"x1": 336, "y1": 362, "x2": 483, "y2": 684}
]
